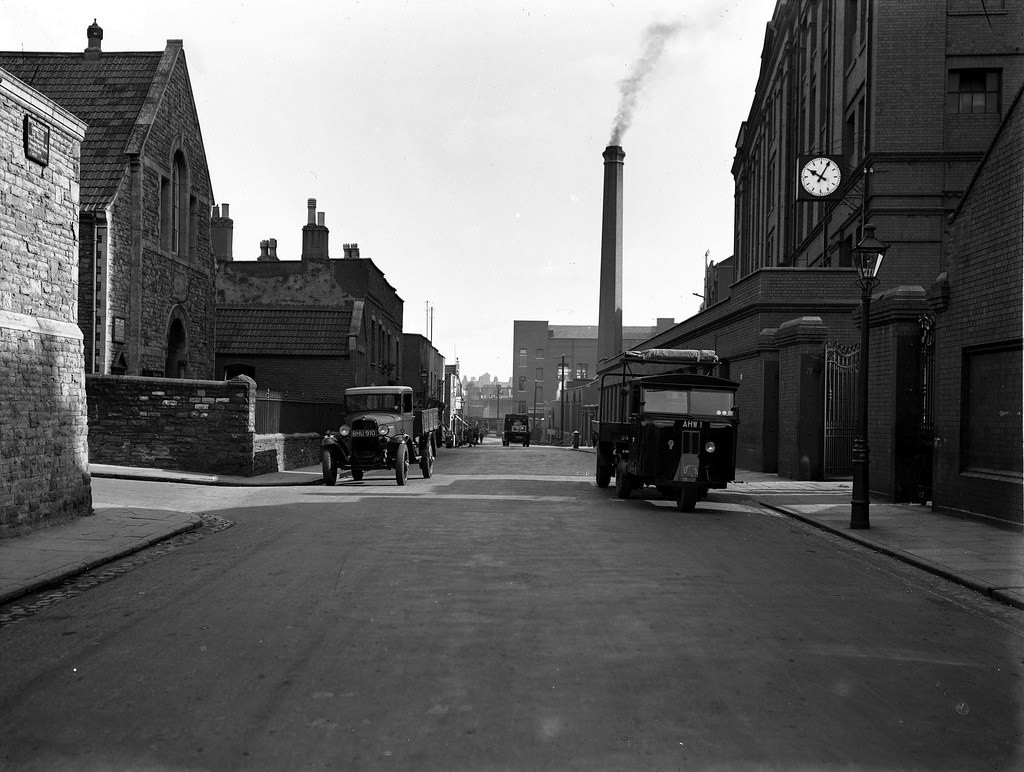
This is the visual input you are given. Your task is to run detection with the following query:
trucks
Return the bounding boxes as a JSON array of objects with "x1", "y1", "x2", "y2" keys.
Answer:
[
  {"x1": 320, "y1": 386, "x2": 441, "y2": 486},
  {"x1": 501, "y1": 414, "x2": 531, "y2": 447}
]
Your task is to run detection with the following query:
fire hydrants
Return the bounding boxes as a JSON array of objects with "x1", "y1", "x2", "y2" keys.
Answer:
[{"x1": 572, "y1": 431, "x2": 580, "y2": 450}]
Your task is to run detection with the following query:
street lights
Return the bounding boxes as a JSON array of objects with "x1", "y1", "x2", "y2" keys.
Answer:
[
  {"x1": 495, "y1": 385, "x2": 502, "y2": 438},
  {"x1": 848, "y1": 231, "x2": 891, "y2": 529},
  {"x1": 561, "y1": 353, "x2": 566, "y2": 446},
  {"x1": 533, "y1": 380, "x2": 539, "y2": 427}
]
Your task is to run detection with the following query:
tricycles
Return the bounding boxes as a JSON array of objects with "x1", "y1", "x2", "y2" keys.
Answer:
[{"x1": 590, "y1": 348, "x2": 741, "y2": 511}]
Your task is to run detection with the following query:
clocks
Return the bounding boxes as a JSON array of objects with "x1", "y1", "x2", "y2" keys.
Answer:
[{"x1": 796, "y1": 154, "x2": 844, "y2": 202}]
